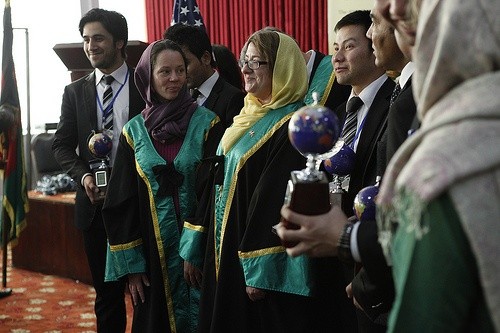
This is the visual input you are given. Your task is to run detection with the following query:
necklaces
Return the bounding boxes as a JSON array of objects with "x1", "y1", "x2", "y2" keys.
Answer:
[{"x1": 349, "y1": 115, "x2": 366, "y2": 150}]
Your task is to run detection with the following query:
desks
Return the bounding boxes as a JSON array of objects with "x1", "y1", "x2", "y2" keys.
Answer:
[{"x1": 11, "y1": 191, "x2": 93, "y2": 286}]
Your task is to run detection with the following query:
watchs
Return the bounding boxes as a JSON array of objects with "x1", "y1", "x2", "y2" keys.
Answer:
[{"x1": 335, "y1": 222, "x2": 354, "y2": 263}]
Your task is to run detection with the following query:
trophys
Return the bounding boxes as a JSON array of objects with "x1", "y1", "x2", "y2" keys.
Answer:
[
  {"x1": 271, "y1": 93, "x2": 381, "y2": 247},
  {"x1": 86, "y1": 130, "x2": 113, "y2": 202}
]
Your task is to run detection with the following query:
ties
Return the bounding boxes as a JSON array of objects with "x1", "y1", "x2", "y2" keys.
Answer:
[
  {"x1": 340, "y1": 97, "x2": 364, "y2": 153},
  {"x1": 101, "y1": 75, "x2": 115, "y2": 141}
]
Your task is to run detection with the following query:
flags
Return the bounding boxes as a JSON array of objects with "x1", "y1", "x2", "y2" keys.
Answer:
[{"x1": 0, "y1": 7, "x2": 30, "y2": 251}]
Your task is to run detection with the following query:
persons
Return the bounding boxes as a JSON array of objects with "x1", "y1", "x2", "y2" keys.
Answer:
[
  {"x1": 102, "y1": 0, "x2": 500, "y2": 333},
  {"x1": 51, "y1": 8, "x2": 147, "y2": 333}
]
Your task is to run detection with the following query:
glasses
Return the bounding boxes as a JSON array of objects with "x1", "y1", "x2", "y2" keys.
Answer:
[{"x1": 237, "y1": 59, "x2": 268, "y2": 70}]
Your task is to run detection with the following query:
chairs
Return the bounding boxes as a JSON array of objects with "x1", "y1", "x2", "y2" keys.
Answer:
[{"x1": 32, "y1": 131, "x2": 62, "y2": 183}]
